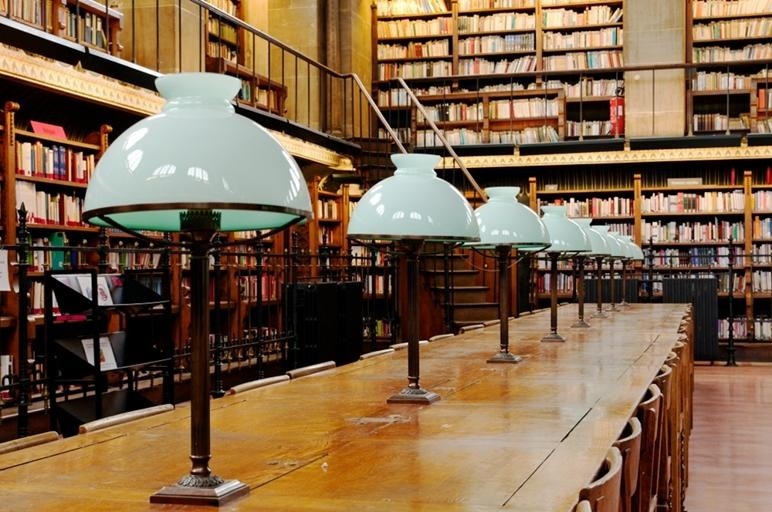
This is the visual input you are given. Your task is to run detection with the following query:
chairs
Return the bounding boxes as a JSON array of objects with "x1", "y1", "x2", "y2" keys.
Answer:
[{"x1": 0, "y1": 304, "x2": 696, "y2": 511}]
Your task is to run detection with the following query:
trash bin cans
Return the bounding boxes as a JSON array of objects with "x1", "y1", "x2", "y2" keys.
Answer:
[
  {"x1": 660, "y1": 278, "x2": 719, "y2": 361},
  {"x1": 584, "y1": 279, "x2": 639, "y2": 303},
  {"x1": 281, "y1": 281, "x2": 365, "y2": 364}
]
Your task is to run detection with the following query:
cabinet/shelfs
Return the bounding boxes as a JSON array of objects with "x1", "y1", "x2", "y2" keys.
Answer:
[
  {"x1": 523, "y1": 157, "x2": 770, "y2": 354},
  {"x1": 369, "y1": 5, "x2": 623, "y2": 138},
  {"x1": 3, "y1": 0, "x2": 125, "y2": 45},
  {"x1": 2, "y1": 77, "x2": 397, "y2": 427},
  {"x1": 684, "y1": 0, "x2": 771, "y2": 134},
  {"x1": 205, "y1": 2, "x2": 246, "y2": 73}
]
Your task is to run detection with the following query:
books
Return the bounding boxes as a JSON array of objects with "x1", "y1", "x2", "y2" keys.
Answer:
[
  {"x1": 541, "y1": 184, "x2": 770, "y2": 340},
  {"x1": 691, "y1": 1, "x2": 772, "y2": 133},
  {"x1": 1, "y1": 0, "x2": 113, "y2": 55},
  {"x1": 1, "y1": 354, "x2": 15, "y2": 394},
  {"x1": 377, "y1": 1, "x2": 622, "y2": 148},
  {"x1": 319, "y1": 180, "x2": 393, "y2": 337},
  {"x1": 207, "y1": 1, "x2": 282, "y2": 116},
  {"x1": 15, "y1": 142, "x2": 165, "y2": 317},
  {"x1": 182, "y1": 229, "x2": 278, "y2": 306}
]
